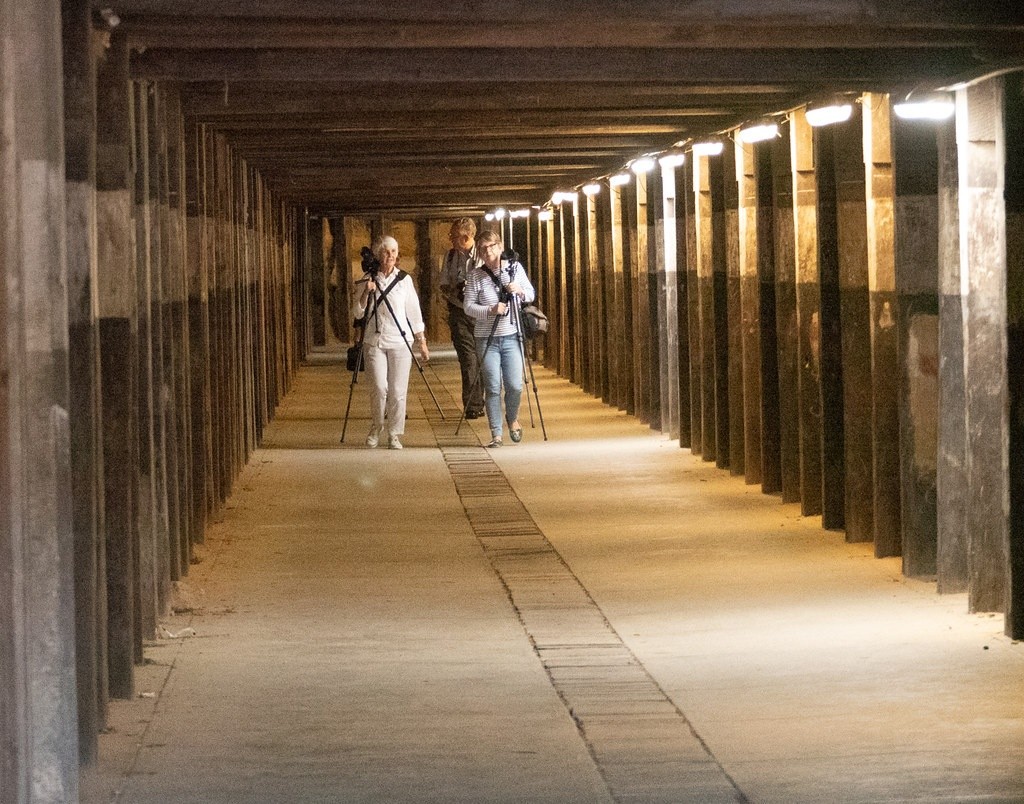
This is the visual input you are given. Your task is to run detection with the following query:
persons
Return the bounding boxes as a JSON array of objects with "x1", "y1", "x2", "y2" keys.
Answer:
[
  {"x1": 463, "y1": 231, "x2": 535, "y2": 449},
  {"x1": 438, "y1": 217, "x2": 485, "y2": 419},
  {"x1": 352, "y1": 235, "x2": 429, "y2": 450}
]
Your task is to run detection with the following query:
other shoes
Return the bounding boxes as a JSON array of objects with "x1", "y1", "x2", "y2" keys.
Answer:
[
  {"x1": 478, "y1": 411, "x2": 485, "y2": 416},
  {"x1": 506, "y1": 415, "x2": 522, "y2": 442},
  {"x1": 489, "y1": 440, "x2": 503, "y2": 448},
  {"x1": 465, "y1": 411, "x2": 478, "y2": 419}
]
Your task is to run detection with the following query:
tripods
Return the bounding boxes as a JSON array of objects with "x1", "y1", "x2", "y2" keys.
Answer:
[
  {"x1": 341, "y1": 272, "x2": 446, "y2": 444},
  {"x1": 453, "y1": 287, "x2": 549, "y2": 440}
]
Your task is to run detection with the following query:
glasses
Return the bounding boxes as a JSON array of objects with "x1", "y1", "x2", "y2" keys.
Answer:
[
  {"x1": 479, "y1": 242, "x2": 497, "y2": 254},
  {"x1": 448, "y1": 233, "x2": 459, "y2": 239}
]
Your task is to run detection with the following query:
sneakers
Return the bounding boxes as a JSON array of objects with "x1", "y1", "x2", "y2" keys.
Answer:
[
  {"x1": 366, "y1": 424, "x2": 384, "y2": 449},
  {"x1": 388, "y1": 436, "x2": 402, "y2": 450}
]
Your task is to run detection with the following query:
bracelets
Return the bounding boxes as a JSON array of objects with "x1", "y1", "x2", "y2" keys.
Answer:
[{"x1": 415, "y1": 337, "x2": 426, "y2": 341}]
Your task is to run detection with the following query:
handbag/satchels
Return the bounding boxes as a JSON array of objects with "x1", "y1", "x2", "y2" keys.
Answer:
[
  {"x1": 522, "y1": 305, "x2": 548, "y2": 339},
  {"x1": 347, "y1": 347, "x2": 364, "y2": 371}
]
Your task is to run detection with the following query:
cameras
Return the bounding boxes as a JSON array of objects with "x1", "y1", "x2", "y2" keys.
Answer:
[
  {"x1": 500, "y1": 249, "x2": 519, "y2": 261},
  {"x1": 360, "y1": 246, "x2": 380, "y2": 272}
]
